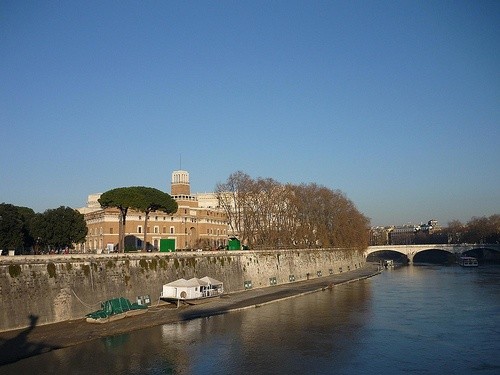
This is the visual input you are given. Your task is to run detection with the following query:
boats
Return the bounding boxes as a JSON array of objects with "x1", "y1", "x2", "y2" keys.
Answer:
[
  {"x1": 160, "y1": 276, "x2": 225, "y2": 308},
  {"x1": 459, "y1": 256, "x2": 479, "y2": 267},
  {"x1": 384, "y1": 259, "x2": 394, "y2": 267},
  {"x1": 86, "y1": 298, "x2": 148, "y2": 324}
]
